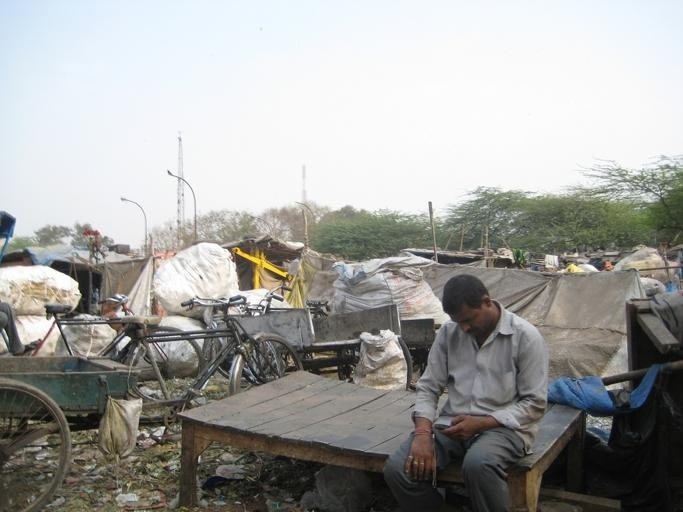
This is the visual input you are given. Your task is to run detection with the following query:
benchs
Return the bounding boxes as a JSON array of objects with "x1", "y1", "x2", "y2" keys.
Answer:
[{"x1": 176, "y1": 368, "x2": 587, "y2": 511}]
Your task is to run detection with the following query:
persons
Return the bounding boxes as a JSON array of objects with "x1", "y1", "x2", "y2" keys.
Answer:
[
  {"x1": 93, "y1": 287, "x2": 100, "y2": 308},
  {"x1": 383, "y1": 272, "x2": 549, "y2": 511},
  {"x1": 603, "y1": 260, "x2": 613, "y2": 271}
]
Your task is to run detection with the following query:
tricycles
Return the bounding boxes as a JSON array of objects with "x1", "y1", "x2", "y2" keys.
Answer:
[
  {"x1": 180, "y1": 285, "x2": 441, "y2": 393},
  {"x1": 0, "y1": 294, "x2": 304, "y2": 512}
]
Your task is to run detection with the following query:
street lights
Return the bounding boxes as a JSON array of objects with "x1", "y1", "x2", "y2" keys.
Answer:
[
  {"x1": 168, "y1": 169, "x2": 197, "y2": 250},
  {"x1": 121, "y1": 197, "x2": 148, "y2": 252}
]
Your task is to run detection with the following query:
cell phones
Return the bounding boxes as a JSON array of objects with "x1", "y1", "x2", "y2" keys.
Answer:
[{"x1": 435, "y1": 424, "x2": 452, "y2": 429}]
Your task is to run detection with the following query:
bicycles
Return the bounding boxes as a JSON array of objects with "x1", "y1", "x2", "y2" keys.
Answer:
[{"x1": 31, "y1": 293, "x2": 208, "y2": 402}]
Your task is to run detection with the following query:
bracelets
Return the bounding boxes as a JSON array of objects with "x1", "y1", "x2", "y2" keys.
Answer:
[{"x1": 414, "y1": 426, "x2": 432, "y2": 434}]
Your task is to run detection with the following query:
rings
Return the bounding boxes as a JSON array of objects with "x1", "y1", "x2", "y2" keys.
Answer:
[
  {"x1": 420, "y1": 463, "x2": 424, "y2": 464},
  {"x1": 413, "y1": 462, "x2": 418, "y2": 464},
  {"x1": 408, "y1": 456, "x2": 414, "y2": 459}
]
